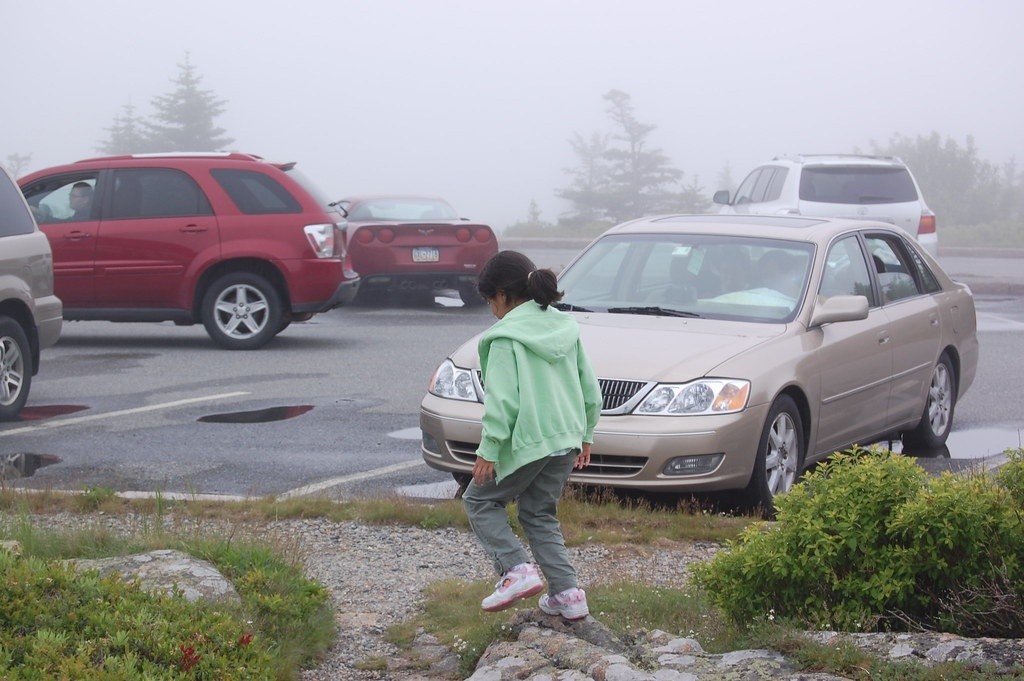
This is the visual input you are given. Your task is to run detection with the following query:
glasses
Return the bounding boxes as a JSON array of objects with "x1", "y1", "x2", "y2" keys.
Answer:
[{"x1": 69, "y1": 193, "x2": 84, "y2": 199}]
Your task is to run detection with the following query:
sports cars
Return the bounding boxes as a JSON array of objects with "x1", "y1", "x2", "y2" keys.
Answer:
[{"x1": 323, "y1": 196, "x2": 499, "y2": 300}]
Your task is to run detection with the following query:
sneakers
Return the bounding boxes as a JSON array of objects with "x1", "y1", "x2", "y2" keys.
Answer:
[
  {"x1": 481, "y1": 562, "x2": 544, "y2": 612},
  {"x1": 538, "y1": 589, "x2": 589, "y2": 619}
]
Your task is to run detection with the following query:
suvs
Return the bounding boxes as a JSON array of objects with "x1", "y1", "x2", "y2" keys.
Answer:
[
  {"x1": 708, "y1": 155, "x2": 938, "y2": 272},
  {"x1": 16, "y1": 150, "x2": 360, "y2": 348}
]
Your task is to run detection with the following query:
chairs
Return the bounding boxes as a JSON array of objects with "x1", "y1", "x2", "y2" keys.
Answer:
[{"x1": 745, "y1": 248, "x2": 804, "y2": 299}]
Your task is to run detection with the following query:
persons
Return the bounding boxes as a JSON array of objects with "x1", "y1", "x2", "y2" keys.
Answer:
[
  {"x1": 462, "y1": 250, "x2": 604, "y2": 619},
  {"x1": 30, "y1": 182, "x2": 93, "y2": 224}
]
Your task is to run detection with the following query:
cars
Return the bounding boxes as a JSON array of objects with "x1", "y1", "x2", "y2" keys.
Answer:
[
  {"x1": 0, "y1": 162, "x2": 63, "y2": 419},
  {"x1": 420, "y1": 214, "x2": 982, "y2": 515}
]
